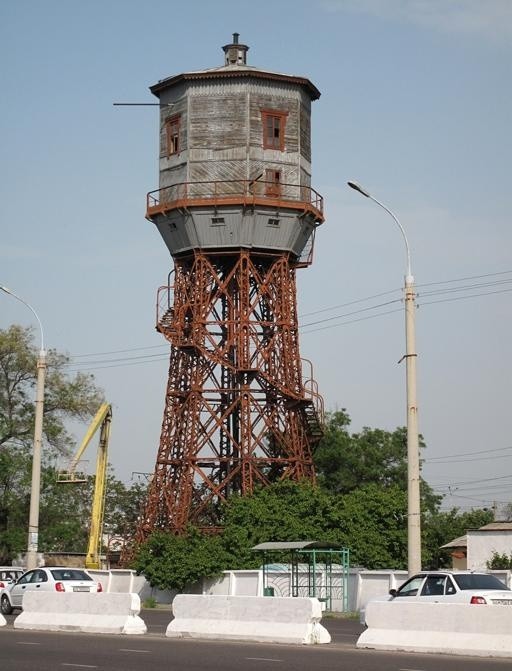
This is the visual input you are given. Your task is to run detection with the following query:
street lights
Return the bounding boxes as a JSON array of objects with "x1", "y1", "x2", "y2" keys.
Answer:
[
  {"x1": 346, "y1": 178, "x2": 422, "y2": 579},
  {"x1": 0, "y1": 283, "x2": 47, "y2": 552}
]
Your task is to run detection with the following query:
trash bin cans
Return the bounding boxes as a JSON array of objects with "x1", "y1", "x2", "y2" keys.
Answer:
[{"x1": 264, "y1": 588, "x2": 274, "y2": 596}]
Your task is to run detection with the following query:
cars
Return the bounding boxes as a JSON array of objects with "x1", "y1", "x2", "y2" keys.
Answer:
[
  {"x1": 359, "y1": 570, "x2": 512, "y2": 630},
  {"x1": 0, "y1": 565, "x2": 104, "y2": 616}
]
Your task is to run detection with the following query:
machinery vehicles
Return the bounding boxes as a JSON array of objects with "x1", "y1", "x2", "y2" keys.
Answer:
[{"x1": 54, "y1": 399, "x2": 113, "y2": 569}]
